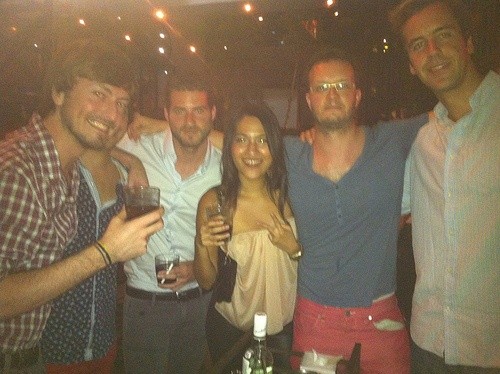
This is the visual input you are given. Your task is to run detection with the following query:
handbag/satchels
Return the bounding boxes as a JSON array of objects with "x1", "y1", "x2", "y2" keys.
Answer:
[{"x1": 210, "y1": 187, "x2": 237, "y2": 303}]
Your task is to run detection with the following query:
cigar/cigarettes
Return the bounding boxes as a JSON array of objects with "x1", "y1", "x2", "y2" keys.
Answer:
[{"x1": 161, "y1": 264, "x2": 174, "y2": 284}]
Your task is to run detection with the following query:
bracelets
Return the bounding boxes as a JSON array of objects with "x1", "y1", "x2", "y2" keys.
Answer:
[
  {"x1": 291, "y1": 251, "x2": 301, "y2": 260},
  {"x1": 95, "y1": 240, "x2": 114, "y2": 265}
]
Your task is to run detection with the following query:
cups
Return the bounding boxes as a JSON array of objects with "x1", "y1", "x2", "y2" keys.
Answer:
[
  {"x1": 155, "y1": 254, "x2": 180, "y2": 287},
  {"x1": 122, "y1": 187, "x2": 160, "y2": 220},
  {"x1": 205, "y1": 206, "x2": 234, "y2": 243}
]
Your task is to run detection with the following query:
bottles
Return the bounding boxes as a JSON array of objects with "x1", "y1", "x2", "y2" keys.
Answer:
[{"x1": 238, "y1": 311, "x2": 275, "y2": 374}]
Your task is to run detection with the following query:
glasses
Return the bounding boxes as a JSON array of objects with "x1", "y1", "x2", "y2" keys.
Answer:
[{"x1": 309, "y1": 79, "x2": 356, "y2": 95}]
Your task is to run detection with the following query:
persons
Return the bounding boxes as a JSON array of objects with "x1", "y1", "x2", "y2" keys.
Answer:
[
  {"x1": 300, "y1": 0, "x2": 500, "y2": 374},
  {"x1": 193, "y1": 106, "x2": 300, "y2": 374},
  {"x1": 112, "y1": 76, "x2": 224, "y2": 374},
  {"x1": 0, "y1": 38, "x2": 164, "y2": 374},
  {"x1": 39, "y1": 105, "x2": 129, "y2": 374},
  {"x1": 128, "y1": 51, "x2": 437, "y2": 374}
]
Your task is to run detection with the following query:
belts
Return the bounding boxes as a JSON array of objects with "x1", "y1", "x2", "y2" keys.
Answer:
[{"x1": 126, "y1": 286, "x2": 205, "y2": 302}]
